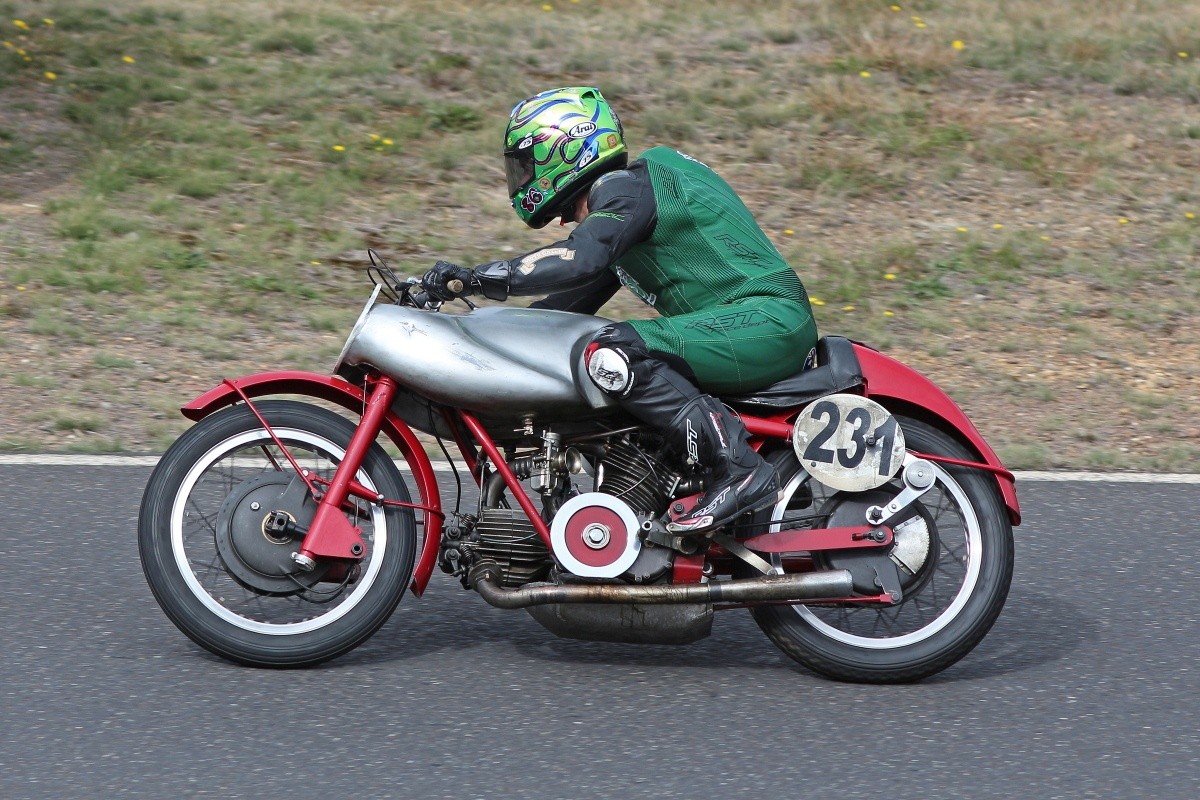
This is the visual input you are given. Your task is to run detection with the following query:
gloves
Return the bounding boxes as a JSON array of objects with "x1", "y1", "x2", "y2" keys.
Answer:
[{"x1": 422, "y1": 260, "x2": 510, "y2": 301}]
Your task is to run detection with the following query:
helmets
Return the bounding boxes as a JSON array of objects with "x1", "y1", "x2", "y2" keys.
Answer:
[{"x1": 504, "y1": 87, "x2": 629, "y2": 229}]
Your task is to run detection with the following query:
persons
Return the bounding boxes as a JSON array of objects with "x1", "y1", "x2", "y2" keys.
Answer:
[{"x1": 422, "y1": 86, "x2": 818, "y2": 532}]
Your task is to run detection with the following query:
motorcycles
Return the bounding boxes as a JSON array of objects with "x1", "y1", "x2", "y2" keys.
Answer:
[{"x1": 136, "y1": 247, "x2": 1023, "y2": 687}]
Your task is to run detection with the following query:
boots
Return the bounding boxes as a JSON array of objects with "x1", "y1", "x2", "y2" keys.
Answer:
[{"x1": 661, "y1": 393, "x2": 785, "y2": 536}]
